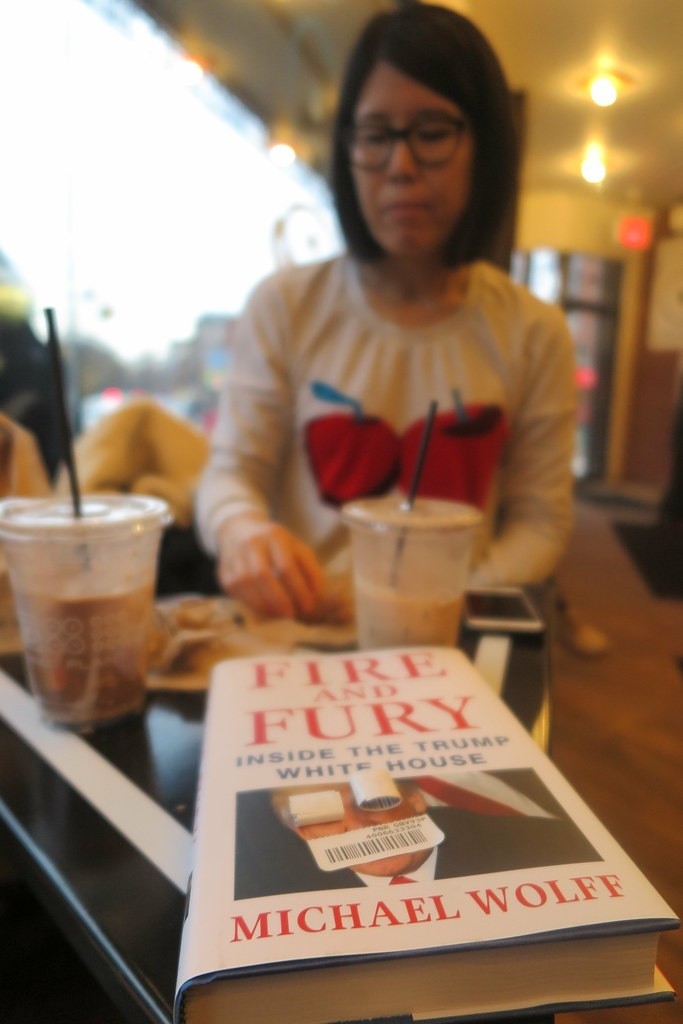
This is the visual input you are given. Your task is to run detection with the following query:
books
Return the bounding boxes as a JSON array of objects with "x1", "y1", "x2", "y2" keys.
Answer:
[{"x1": 171, "y1": 645, "x2": 680, "y2": 1023}]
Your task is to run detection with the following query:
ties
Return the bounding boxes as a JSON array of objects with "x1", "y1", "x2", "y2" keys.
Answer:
[{"x1": 391, "y1": 876, "x2": 416, "y2": 883}]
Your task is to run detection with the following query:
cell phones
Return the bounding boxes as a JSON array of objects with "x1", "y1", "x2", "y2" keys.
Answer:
[{"x1": 462, "y1": 587, "x2": 543, "y2": 635}]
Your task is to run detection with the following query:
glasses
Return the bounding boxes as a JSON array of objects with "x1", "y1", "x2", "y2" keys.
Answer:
[{"x1": 345, "y1": 113, "x2": 477, "y2": 170}]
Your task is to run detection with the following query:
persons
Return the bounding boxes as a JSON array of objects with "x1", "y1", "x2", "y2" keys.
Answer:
[
  {"x1": 235, "y1": 779, "x2": 600, "y2": 900},
  {"x1": 194, "y1": 0, "x2": 581, "y2": 624}
]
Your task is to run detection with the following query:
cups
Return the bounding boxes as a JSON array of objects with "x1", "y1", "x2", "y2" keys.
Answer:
[
  {"x1": 341, "y1": 497, "x2": 485, "y2": 647},
  {"x1": 0, "y1": 494, "x2": 173, "y2": 726}
]
